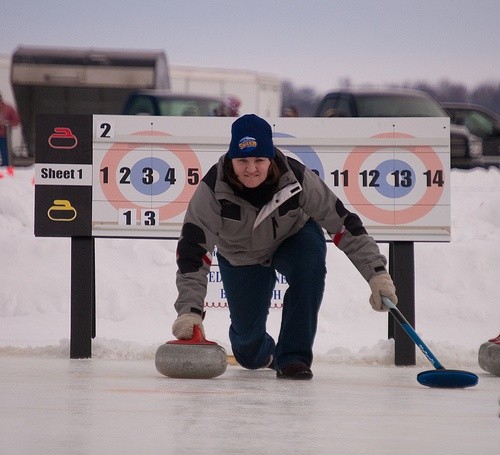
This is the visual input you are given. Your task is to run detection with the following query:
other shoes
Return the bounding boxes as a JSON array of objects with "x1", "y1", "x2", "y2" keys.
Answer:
[{"x1": 276, "y1": 362, "x2": 313, "y2": 381}]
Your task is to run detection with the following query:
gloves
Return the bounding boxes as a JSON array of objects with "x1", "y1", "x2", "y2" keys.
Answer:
[
  {"x1": 172, "y1": 313, "x2": 205, "y2": 340},
  {"x1": 369, "y1": 274, "x2": 398, "y2": 312}
]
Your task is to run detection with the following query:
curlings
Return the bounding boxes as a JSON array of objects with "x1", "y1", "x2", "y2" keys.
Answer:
[
  {"x1": 154, "y1": 326, "x2": 228, "y2": 379},
  {"x1": 477, "y1": 334, "x2": 500, "y2": 377}
]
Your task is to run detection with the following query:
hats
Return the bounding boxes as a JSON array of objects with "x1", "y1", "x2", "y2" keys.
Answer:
[
  {"x1": 223, "y1": 95, "x2": 240, "y2": 112},
  {"x1": 225, "y1": 114, "x2": 276, "y2": 162},
  {"x1": 325, "y1": 109, "x2": 336, "y2": 116}
]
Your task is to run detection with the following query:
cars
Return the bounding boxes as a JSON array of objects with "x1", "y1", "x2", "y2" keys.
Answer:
[
  {"x1": 120, "y1": 88, "x2": 240, "y2": 117},
  {"x1": 314, "y1": 87, "x2": 483, "y2": 161},
  {"x1": 439, "y1": 101, "x2": 500, "y2": 156}
]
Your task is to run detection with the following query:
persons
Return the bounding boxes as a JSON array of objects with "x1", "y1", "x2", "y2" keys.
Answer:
[
  {"x1": 283, "y1": 106, "x2": 297, "y2": 116},
  {"x1": 0, "y1": 95, "x2": 20, "y2": 166},
  {"x1": 171, "y1": 114, "x2": 399, "y2": 380}
]
